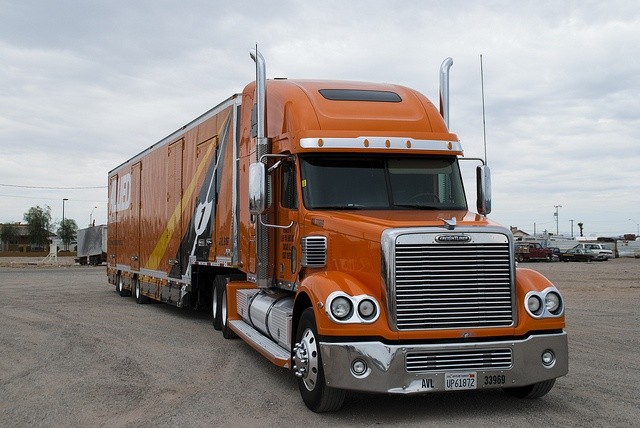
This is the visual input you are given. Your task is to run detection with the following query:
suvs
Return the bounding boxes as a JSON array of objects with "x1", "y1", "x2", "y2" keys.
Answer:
[{"x1": 515, "y1": 241, "x2": 552, "y2": 262}]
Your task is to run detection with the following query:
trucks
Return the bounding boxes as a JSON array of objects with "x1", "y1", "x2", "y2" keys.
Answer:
[
  {"x1": 76, "y1": 223, "x2": 107, "y2": 265},
  {"x1": 106, "y1": 43, "x2": 569, "y2": 413}
]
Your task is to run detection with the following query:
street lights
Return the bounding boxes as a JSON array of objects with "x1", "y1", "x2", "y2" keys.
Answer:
[{"x1": 62, "y1": 198, "x2": 69, "y2": 243}]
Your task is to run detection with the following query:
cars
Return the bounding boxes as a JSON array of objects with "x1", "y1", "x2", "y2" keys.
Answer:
[
  {"x1": 560, "y1": 247, "x2": 598, "y2": 262},
  {"x1": 549, "y1": 246, "x2": 574, "y2": 261}
]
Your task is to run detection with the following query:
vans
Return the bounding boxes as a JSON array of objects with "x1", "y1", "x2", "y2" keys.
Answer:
[{"x1": 573, "y1": 243, "x2": 613, "y2": 261}]
